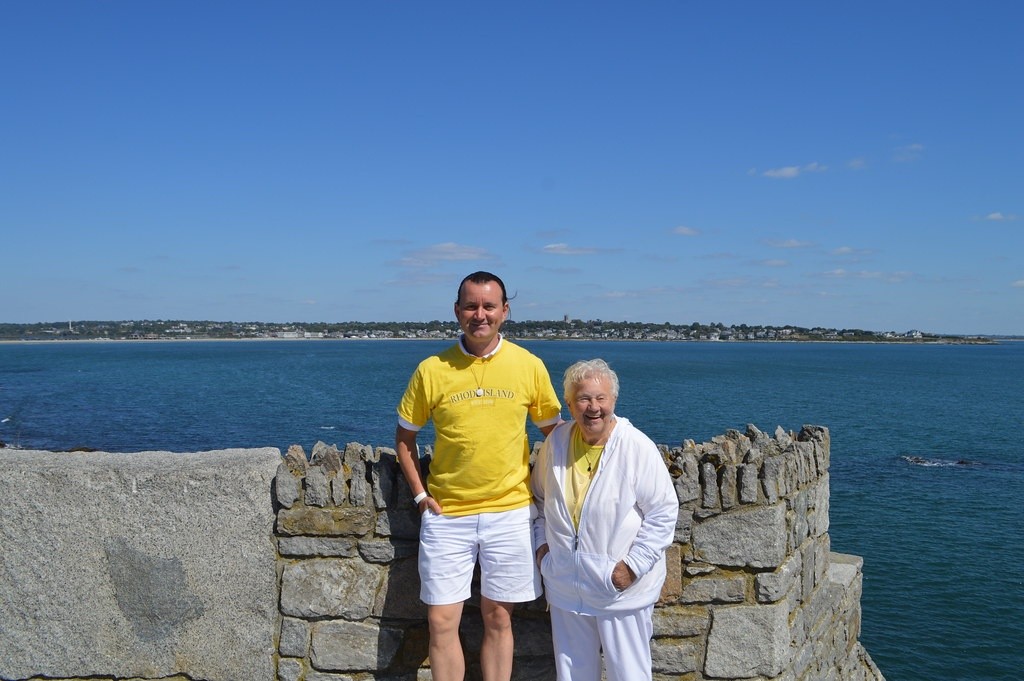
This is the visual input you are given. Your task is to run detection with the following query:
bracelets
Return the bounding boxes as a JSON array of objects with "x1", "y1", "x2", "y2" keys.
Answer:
[{"x1": 414, "y1": 491, "x2": 430, "y2": 504}]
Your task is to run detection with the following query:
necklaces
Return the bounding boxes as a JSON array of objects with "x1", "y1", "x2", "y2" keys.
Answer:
[
  {"x1": 468, "y1": 351, "x2": 491, "y2": 396},
  {"x1": 581, "y1": 431, "x2": 610, "y2": 471}
]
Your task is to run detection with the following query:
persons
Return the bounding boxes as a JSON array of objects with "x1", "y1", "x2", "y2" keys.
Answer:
[
  {"x1": 395, "y1": 271, "x2": 566, "y2": 681},
  {"x1": 531, "y1": 358, "x2": 679, "y2": 681}
]
